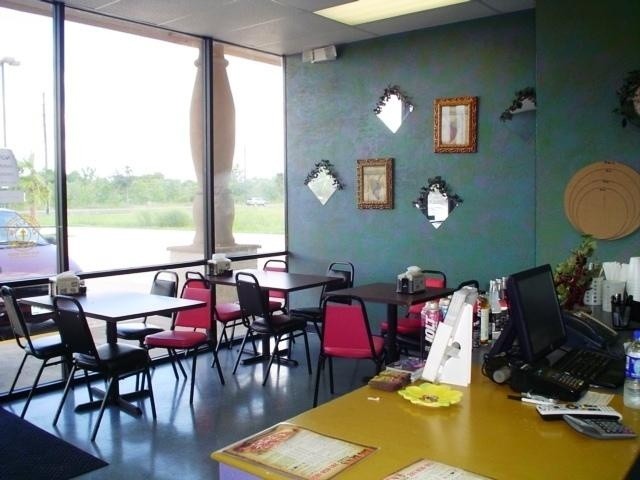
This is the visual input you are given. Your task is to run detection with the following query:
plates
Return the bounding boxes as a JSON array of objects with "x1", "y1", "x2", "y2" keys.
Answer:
[{"x1": 398, "y1": 382, "x2": 463, "y2": 409}]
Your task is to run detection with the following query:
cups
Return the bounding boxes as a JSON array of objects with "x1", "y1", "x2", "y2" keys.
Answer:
[
  {"x1": 625, "y1": 256, "x2": 639, "y2": 303},
  {"x1": 612, "y1": 301, "x2": 631, "y2": 329},
  {"x1": 602, "y1": 279, "x2": 626, "y2": 313},
  {"x1": 581, "y1": 277, "x2": 604, "y2": 305}
]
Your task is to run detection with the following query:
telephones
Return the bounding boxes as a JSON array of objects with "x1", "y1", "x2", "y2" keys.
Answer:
[{"x1": 562, "y1": 310, "x2": 617, "y2": 349}]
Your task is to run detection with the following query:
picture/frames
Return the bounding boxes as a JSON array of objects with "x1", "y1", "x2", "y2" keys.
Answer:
[
  {"x1": 434, "y1": 95, "x2": 478, "y2": 154},
  {"x1": 355, "y1": 157, "x2": 394, "y2": 210}
]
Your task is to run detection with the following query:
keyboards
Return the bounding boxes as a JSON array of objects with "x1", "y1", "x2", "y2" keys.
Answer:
[{"x1": 547, "y1": 346, "x2": 613, "y2": 395}]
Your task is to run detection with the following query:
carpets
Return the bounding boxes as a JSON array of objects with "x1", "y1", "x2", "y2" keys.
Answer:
[{"x1": 0, "y1": 404, "x2": 110, "y2": 479}]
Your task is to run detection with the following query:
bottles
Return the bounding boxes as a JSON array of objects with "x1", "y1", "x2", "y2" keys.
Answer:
[
  {"x1": 490, "y1": 277, "x2": 509, "y2": 340},
  {"x1": 420, "y1": 292, "x2": 490, "y2": 360},
  {"x1": 624, "y1": 330, "x2": 640, "y2": 408}
]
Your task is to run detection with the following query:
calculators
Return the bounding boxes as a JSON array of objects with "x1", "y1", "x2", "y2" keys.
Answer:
[{"x1": 562, "y1": 415, "x2": 638, "y2": 440}]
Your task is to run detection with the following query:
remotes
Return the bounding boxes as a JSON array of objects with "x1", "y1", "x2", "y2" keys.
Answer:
[{"x1": 536, "y1": 404, "x2": 623, "y2": 421}]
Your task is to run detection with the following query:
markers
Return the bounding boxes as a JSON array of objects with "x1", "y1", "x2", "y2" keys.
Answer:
[{"x1": 611, "y1": 291, "x2": 633, "y2": 304}]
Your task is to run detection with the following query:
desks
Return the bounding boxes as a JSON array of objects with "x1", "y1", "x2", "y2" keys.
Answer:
[{"x1": 211, "y1": 344, "x2": 640, "y2": 480}]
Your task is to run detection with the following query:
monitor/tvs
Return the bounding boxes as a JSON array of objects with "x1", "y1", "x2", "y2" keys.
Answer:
[{"x1": 488, "y1": 263, "x2": 569, "y2": 371}]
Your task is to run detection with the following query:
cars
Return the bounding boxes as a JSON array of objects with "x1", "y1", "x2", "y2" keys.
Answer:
[{"x1": 1, "y1": 210, "x2": 89, "y2": 310}]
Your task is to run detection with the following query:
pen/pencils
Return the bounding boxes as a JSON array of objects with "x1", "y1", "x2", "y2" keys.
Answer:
[{"x1": 507, "y1": 394, "x2": 542, "y2": 404}]
